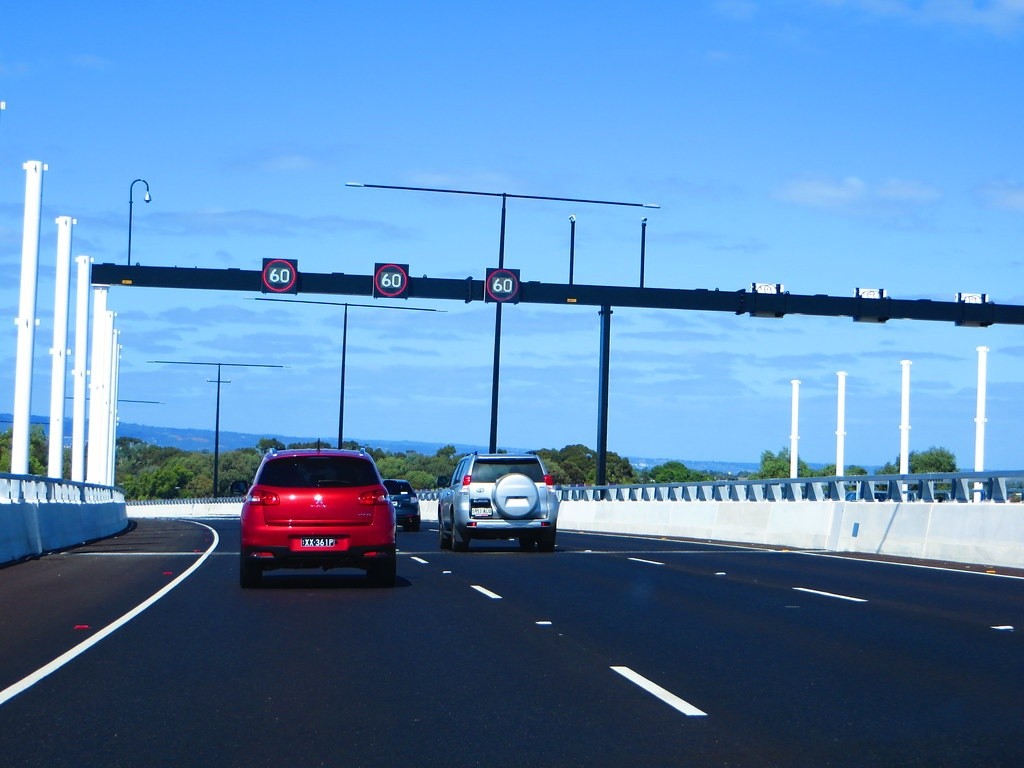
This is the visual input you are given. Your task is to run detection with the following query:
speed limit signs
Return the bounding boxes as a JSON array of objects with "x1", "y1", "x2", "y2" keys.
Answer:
[
  {"x1": 484, "y1": 268, "x2": 521, "y2": 305},
  {"x1": 373, "y1": 262, "x2": 409, "y2": 299},
  {"x1": 261, "y1": 258, "x2": 297, "y2": 296}
]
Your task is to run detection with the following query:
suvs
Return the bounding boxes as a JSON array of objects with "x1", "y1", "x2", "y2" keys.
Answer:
[
  {"x1": 240, "y1": 448, "x2": 398, "y2": 589},
  {"x1": 436, "y1": 451, "x2": 560, "y2": 552},
  {"x1": 380, "y1": 479, "x2": 421, "y2": 531}
]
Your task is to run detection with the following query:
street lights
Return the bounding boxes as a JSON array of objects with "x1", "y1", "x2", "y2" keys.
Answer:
[
  {"x1": 69, "y1": 255, "x2": 94, "y2": 486},
  {"x1": 973, "y1": 346, "x2": 990, "y2": 504},
  {"x1": 899, "y1": 360, "x2": 913, "y2": 502},
  {"x1": 346, "y1": 181, "x2": 661, "y2": 455},
  {"x1": 254, "y1": 298, "x2": 449, "y2": 451},
  {"x1": 89, "y1": 283, "x2": 124, "y2": 489},
  {"x1": 47, "y1": 216, "x2": 76, "y2": 480},
  {"x1": 127, "y1": 177, "x2": 151, "y2": 265},
  {"x1": 146, "y1": 359, "x2": 291, "y2": 501},
  {"x1": 835, "y1": 370, "x2": 848, "y2": 501},
  {"x1": 790, "y1": 379, "x2": 802, "y2": 479},
  {"x1": 11, "y1": 160, "x2": 49, "y2": 472}
]
